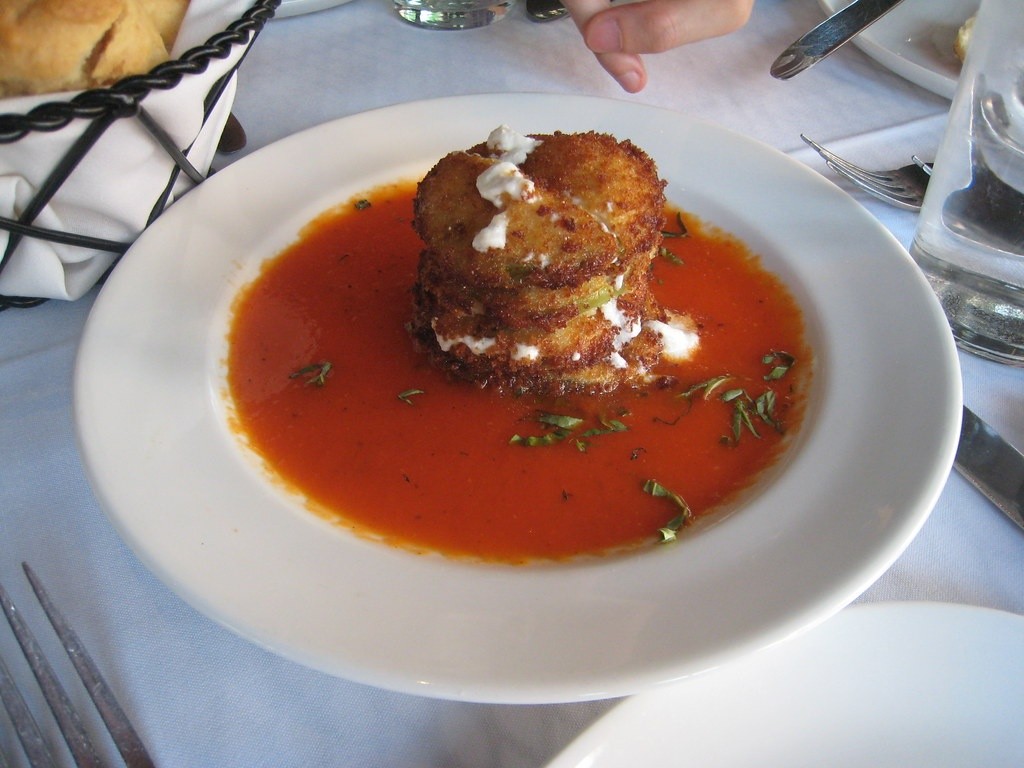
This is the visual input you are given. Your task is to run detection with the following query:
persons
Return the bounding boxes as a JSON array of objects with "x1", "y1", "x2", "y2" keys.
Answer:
[{"x1": 559, "y1": 0, "x2": 756, "y2": 93}]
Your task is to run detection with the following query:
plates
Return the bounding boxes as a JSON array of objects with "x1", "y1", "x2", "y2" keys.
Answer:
[
  {"x1": 74, "y1": 92, "x2": 952, "y2": 705},
  {"x1": 819, "y1": 0, "x2": 982, "y2": 99},
  {"x1": 540, "y1": 597, "x2": 1022, "y2": 766}
]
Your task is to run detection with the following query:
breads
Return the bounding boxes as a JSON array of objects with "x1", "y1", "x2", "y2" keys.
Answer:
[{"x1": 0, "y1": 0, "x2": 193, "y2": 99}]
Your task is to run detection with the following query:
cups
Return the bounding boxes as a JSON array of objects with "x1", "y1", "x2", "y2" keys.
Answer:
[
  {"x1": 394, "y1": 1, "x2": 514, "y2": 29},
  {"x1": 904, "y1": 1, "x2": 1022, "y2": 363}
]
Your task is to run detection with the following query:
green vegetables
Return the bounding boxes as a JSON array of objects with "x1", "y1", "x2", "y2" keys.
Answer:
[{"x1": 290, "y1": 199, "x2": 794, "y2": 544}]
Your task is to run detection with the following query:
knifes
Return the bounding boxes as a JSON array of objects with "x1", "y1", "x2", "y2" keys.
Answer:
[
  {"x1": 951, "y1": 404, "x2": 1024, "y2": 533},
  {"x1": 771, "y1": 2, "x2": 904, "y2": 80}
]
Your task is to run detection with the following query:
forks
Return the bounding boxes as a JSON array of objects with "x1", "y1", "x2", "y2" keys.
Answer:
[
  {"x1": 0, "y1": 563, "x2": 155, "y2": 768},
  {"x1": 797, "y1": 132, "x2": 934, "y2": 212}
]
